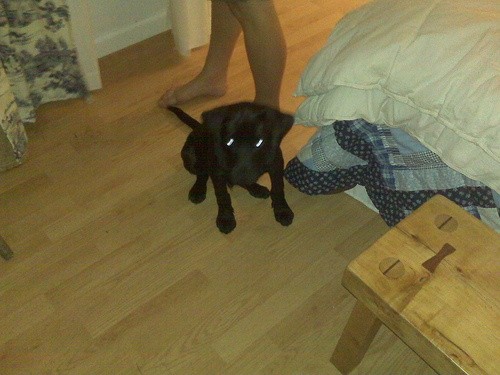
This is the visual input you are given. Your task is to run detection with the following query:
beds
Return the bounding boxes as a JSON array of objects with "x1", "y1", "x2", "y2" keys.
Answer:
[{"x1": 284, "y1": 1, "x2": 499, "y2": 236}]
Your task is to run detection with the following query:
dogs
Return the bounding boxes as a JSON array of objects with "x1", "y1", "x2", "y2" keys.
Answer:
[{"x1": 167, "y1": 101, "x2": 295, "y2": 233}]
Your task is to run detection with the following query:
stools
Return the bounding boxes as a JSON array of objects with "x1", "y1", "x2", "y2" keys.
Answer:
[{"x1": 330, "y1": 194, "x2": 500, "y2": 374}]
